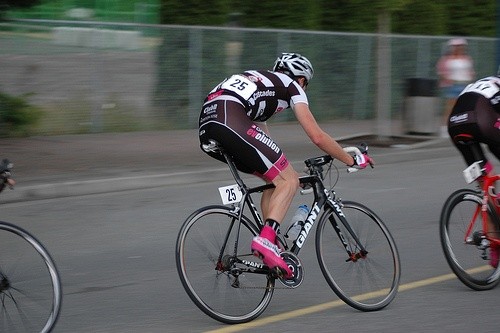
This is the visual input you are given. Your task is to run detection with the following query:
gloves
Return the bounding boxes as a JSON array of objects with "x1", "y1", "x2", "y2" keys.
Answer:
[{"x1": 349, "y1": 153, "x2": 374, "y2": 168}]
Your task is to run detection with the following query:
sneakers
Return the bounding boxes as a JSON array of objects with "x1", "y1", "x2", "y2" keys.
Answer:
[{"x1": 250, "y1": 235, "x2": 292, "y2": 279}]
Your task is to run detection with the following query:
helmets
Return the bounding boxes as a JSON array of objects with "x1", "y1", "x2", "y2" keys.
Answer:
[{"x1": 273, "y1": 52, "x2": 314, "y2": 83}]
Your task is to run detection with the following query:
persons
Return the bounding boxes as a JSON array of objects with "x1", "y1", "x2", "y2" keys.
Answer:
[
  {"x1": 436, "y1": 37, "x2": 474, "y2": 129},
  {"x1": 448, "y1": 66, "x2": 500, "y2": 268},
  {"x1": 199, "y1": 53, "x2": 374, "y2": 280}
]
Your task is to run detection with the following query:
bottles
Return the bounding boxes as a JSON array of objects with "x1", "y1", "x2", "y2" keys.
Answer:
[{"x1": 285, "y1": 205, "x2": 310, "y2": 239}]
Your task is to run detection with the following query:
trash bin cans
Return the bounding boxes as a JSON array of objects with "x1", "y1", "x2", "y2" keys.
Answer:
[{"x1": 402, "y1": 67, "x2": 440, "y2": 135}]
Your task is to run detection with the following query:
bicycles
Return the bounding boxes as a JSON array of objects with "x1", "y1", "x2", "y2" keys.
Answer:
[
  {"x1": 0, "y1": 159, "x2": 62, "y2": 333},
  {"x1": 438, "y1": 133, "x2": 500, "y2": 291},
  {"x1": 174, "y1": 138, "x2": 402, "y2": 325}
]
